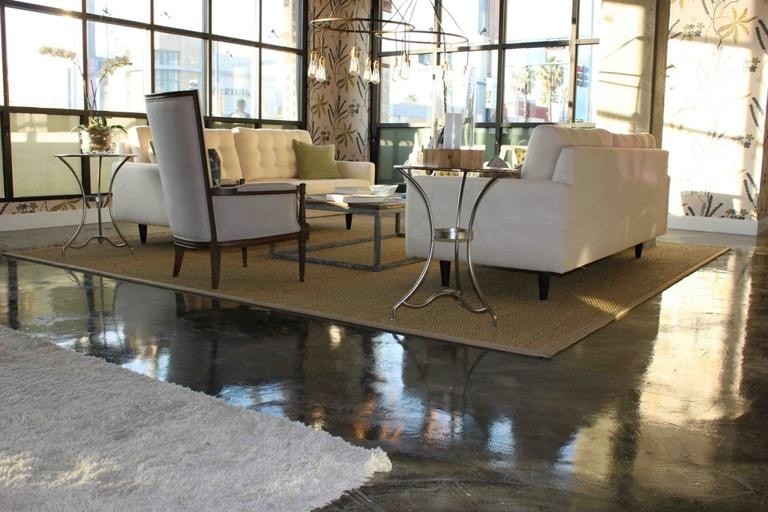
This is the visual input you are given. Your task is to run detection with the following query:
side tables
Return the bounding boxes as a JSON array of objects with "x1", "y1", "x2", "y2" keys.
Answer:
[
  {"x1": 392, "y1": 164, "x2": 520, "y2": 329},
  {"x1": 53, "y1": 153, "x2": 136, "y2": 256}
]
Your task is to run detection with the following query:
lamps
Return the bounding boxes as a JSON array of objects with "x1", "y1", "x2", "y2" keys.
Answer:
[
  {"x1": 349, "y1": 47, "x2": 360, "y2": 76},
  {"x1": 390, "y1": 58, "x2": 400, "y2": 82},
  {"x1": 315, "y1": 57, "x2": 326, "y2": 83},
  {"x1": 363, "y1": 57, "x2": 372, "y2": 82},
  {"x1": 307, "y1": 51, "x2": 318, "y2": 78},
  {"x1": 401, "y1": 52, "x2": 411, "y2": 79},
  {"x1": 372, "y1": 60, "x2": 380, "y2": 84}
]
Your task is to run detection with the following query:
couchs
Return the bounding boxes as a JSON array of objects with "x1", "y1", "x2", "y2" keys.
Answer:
[
  {"x1": 403, "y1": 125, "x2": 671, "y2": 301},
  {"x1": 111, "y1": 124, "x2": 375, "y2": 244}
]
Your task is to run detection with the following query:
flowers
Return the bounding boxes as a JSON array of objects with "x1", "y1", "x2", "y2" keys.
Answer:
[{"x1": 40, "y1": 46, "x2": 130, "y2": 134}]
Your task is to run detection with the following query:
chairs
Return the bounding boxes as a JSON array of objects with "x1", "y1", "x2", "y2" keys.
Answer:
[{"x1": 143, "y1": 89, "x2": 311, "y2": 289}]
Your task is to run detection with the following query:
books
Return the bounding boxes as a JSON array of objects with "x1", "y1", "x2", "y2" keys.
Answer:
[{"x1": 335, "y1": 184, "x2": 401, "y2": 204}]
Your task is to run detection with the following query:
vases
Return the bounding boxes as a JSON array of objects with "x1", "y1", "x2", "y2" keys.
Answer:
[{"x1": 80, "y1": 130, "x2": 114, "y2": 154}]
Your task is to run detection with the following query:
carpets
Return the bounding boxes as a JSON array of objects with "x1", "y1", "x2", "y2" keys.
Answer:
[
  {"x1": 1, "y1": 325, "x2": 345, "y2": 441},
  {"x1": 2, "y1": 207, "x2": 707, "y2": 357}
]
